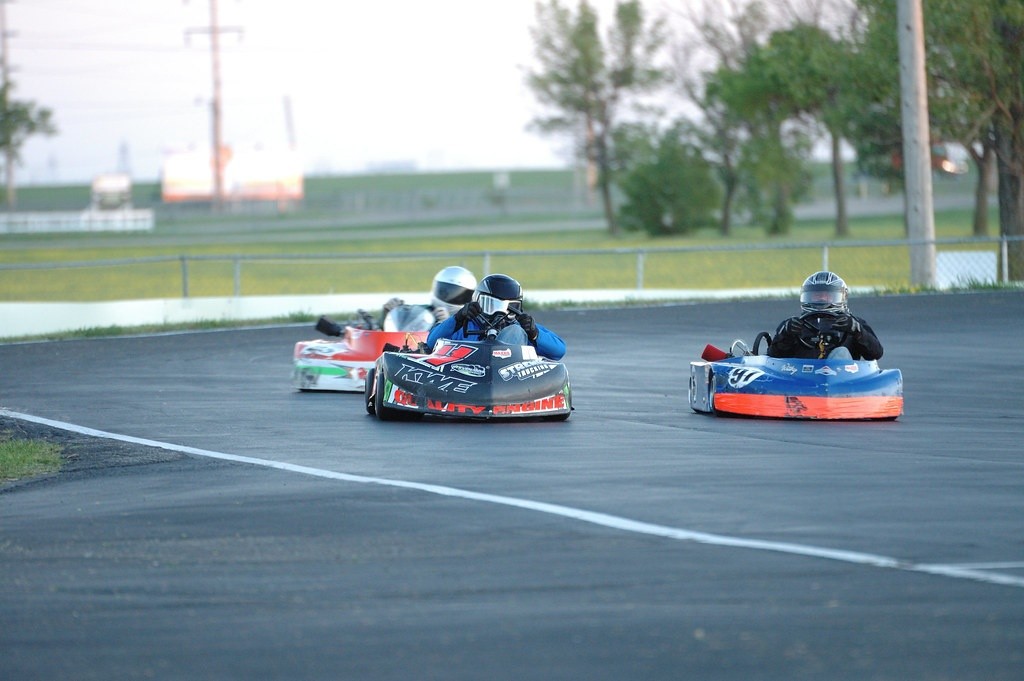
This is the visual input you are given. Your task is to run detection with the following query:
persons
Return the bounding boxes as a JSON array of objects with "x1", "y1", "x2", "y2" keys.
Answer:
[
  {"x1": 426, "y1": 273, "x2": 566, "y2": 361},
  {"x1": 767, "y1": 271, "x2": 883, "y2": 361},
  {"x1": 379, "y1": 265, "x2": 478, "y2": 333}
]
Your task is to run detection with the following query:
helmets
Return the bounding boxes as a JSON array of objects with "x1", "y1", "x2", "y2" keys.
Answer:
[
  {"x1": 800, "y1": 271, "x2": 848, "y2": 314},
  {"x1": 430, "y1": 266, "x2": 477, "y2": 316},
  {"x1": 471, "y1": 274, "x2": 523, "y2": 320}
]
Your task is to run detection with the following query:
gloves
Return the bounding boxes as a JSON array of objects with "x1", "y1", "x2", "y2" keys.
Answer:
[
  {"x1": 455, "y1": 301, "x2": 484, "y2": 331},
  {"x1": 784, "y1": 317, "x2": 804, "y2": 337},
  {"x1": 515, "y1": 312, "x2": 537, "y2": 341},
  {"x1": 831, "y1": 315, "x2": 861, "y2": 335}
]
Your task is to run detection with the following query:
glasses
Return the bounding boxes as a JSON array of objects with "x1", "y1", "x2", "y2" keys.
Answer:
[{"x1": 477, "y1": 294, "x2": 523, "y2": 320}]
